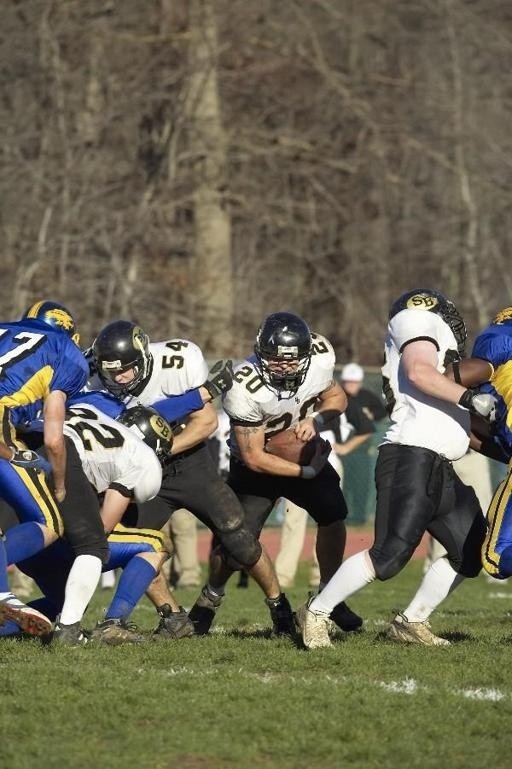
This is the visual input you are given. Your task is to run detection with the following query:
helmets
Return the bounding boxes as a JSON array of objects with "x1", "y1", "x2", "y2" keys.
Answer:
[
  {"x1": 22, "y1": 299, "x2": 153, "y2": 396},
  {"x1": 389, "y1": 288, "x2": 512, "y2": 357},
  {"x1": 253, "y1": 313, "x2": 311, "y2": 391},
  {"x1": 114, "y1": 406, "x2": 173, "y2": 461}
]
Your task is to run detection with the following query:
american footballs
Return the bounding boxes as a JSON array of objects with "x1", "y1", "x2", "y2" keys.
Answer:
[
  {"x1": 263, "y1": 431, "x2": 328, "y2": 467},
  {"x1": 444, "y1": 359, "x2": 495, "y2": 386}
]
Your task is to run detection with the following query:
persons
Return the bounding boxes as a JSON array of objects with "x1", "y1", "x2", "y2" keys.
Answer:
[
  {"x1": 274, "y1": 362, "x2": 387, "y2": 591},
  {"x1": 292, "y1": 288, "x2": 504, "y2": 649},
  {"x1": 445, "y1": 306, "x2": 512, "y2": 581},
  {"x1": 1, "y1": 300, "x2": 362, "y2": 650}
]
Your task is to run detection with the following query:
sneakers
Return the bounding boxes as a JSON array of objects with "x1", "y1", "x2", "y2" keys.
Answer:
[
  {"x1": 265, "y1": 591, "x2": 362, "y2": 650},
  {"x1": 148, "y1": 585, "x2": 224, "y2": 642},
  {"x1": 386, "y1": 609, "x2": 453, "y2": 649},
  {"x1": 0, "y1": 591, "x2": 146, "y2": 649}
]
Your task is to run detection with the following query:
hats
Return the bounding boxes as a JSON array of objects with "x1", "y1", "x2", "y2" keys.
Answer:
[{"x1": 340, "y1": 362, "x2": 365, "y2": 381}]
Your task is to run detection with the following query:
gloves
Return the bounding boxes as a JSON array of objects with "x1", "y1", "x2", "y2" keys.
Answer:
[
  {"x1": 457, "y1": 389, "x2": 502, "y2": 425},
  {"x1": 8, "y1": 445, "x2": 53, "y2": 477},
  {"x1": 203, "y1": 360, "x2": 233, "y2": 399}
]
